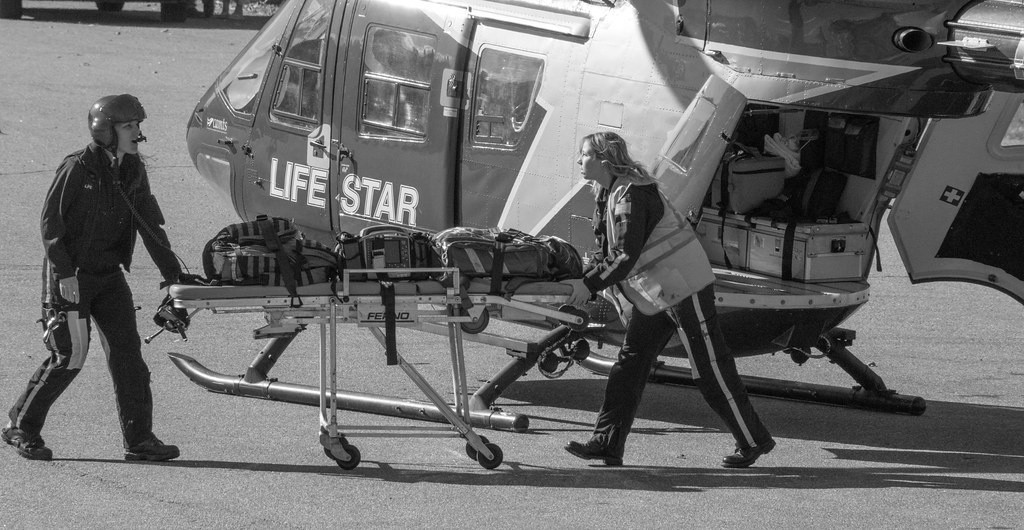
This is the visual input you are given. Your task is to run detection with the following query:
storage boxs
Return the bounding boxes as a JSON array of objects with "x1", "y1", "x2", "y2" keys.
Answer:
[
  {"x1": 694, "y1": 205, "x2": 748, "y2": 269},
  {"x1": 711, "y1": 155, "x2": 784, "y2": 214},
  {"x1": 746, "y1": 215, "x2": 869, "y2": 283}
]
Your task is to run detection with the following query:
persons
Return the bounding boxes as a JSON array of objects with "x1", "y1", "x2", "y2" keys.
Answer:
[
  {"x1": 558, "y1": 132, "x2": 776, "y2": 468},
  {"x1": 0, "y1": 94, "x2": 186, "y2": 460}
]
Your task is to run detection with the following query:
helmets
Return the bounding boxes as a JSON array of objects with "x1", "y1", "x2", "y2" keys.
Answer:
[{"x1": 88, "y1": 94, "x2": 146, "y2": 151}]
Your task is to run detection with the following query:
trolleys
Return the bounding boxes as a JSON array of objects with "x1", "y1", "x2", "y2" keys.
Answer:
[{"x1": 170, "y1": 267, "x2": 598, "y2": 470}]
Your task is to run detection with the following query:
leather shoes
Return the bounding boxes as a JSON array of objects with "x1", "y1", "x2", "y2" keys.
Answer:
[
  {"x1": 722, "y1": 440, "x2": 777, "y2": 466},
  {"x1": 565, "y1": 441, "x2": 623, "y2": 466}
]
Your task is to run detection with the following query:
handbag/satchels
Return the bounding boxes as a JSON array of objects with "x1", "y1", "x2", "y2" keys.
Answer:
[{"x1": 711, "y1": 140, "x2": 786, "y2": 214}]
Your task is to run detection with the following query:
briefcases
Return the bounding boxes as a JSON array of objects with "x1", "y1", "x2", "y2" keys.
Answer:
[{"x1": 338, "y1": 224, "x2": 435, "y2": 283}]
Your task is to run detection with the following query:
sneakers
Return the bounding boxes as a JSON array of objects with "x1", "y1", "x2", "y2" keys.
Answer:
[
  {"x1": 2, "y1": 420, "x2": 52, "y2": 461},
  {"x1": 124, "y1": 435, "x2": 180, "y2": 460}
]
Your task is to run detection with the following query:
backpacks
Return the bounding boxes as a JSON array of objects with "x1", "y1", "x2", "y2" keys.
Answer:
[
  {"x1": 438, "y1": 225, "x2": 552, "y2": 275},
  {"x1": 208, "y1": 217, "x2": 338, "y2": 283}
]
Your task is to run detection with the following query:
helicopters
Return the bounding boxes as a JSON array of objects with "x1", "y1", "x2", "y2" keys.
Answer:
[{"x1": 169, "y1": 1, "x2": 1023, "y2": 430}]
